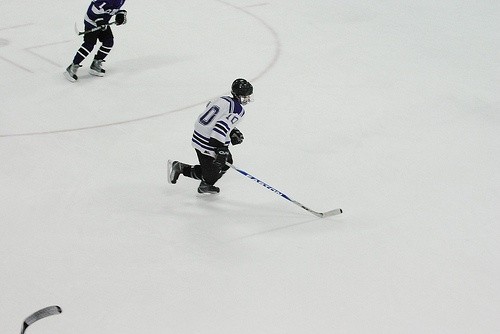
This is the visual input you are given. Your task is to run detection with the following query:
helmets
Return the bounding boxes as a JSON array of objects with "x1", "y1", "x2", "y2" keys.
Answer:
[{"x1": 231, "y1": 78, "x2": 253, "y2": 95}]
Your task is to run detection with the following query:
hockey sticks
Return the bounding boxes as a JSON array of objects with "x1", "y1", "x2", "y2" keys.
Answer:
[
  {"x1": 74, "y1": 21, "x2": 115, "y2": 36},
  {"x1": 224, "y1": 161, "x2": 343, "y2": 219},
  {"x1": 19, "y1": 304, "x2": 62, "y2": 334}
]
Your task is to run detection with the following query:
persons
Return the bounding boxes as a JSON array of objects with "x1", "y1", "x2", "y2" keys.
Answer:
[
  {"x1": 66, "y1": 0, "x2": 127, "y2": 81},
  {"x1": 170, "y1": 79, "x2": 253, "y2": 194}
]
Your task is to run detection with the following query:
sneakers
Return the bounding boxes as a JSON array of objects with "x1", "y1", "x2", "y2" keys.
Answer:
[
  {"x1": 64, "y1": 61, "x2": 83, "y2": 82},
  {"x1": 197, "y1": 179, "x2": 220, "y2": 194},
  {"x1": 89, "y1": 57, "x2": 106, "y2": 77},
  {"x1": 167, "y1": 159, "x2": 191, "y2": 184}
]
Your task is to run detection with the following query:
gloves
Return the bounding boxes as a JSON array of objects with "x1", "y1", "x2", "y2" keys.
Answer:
[
  {"x1": 95, "y1": 17, "x2": 110, "y2": 32},
  {"x1": 216, "y1": 148, "x2": 230, "y2": 173},
  {"x1": 115, "y1": 10, "x2": 127, "y2": 25},
  {"x1": 230, "y1": 127, "x2": 244, "y2": 145}
]
